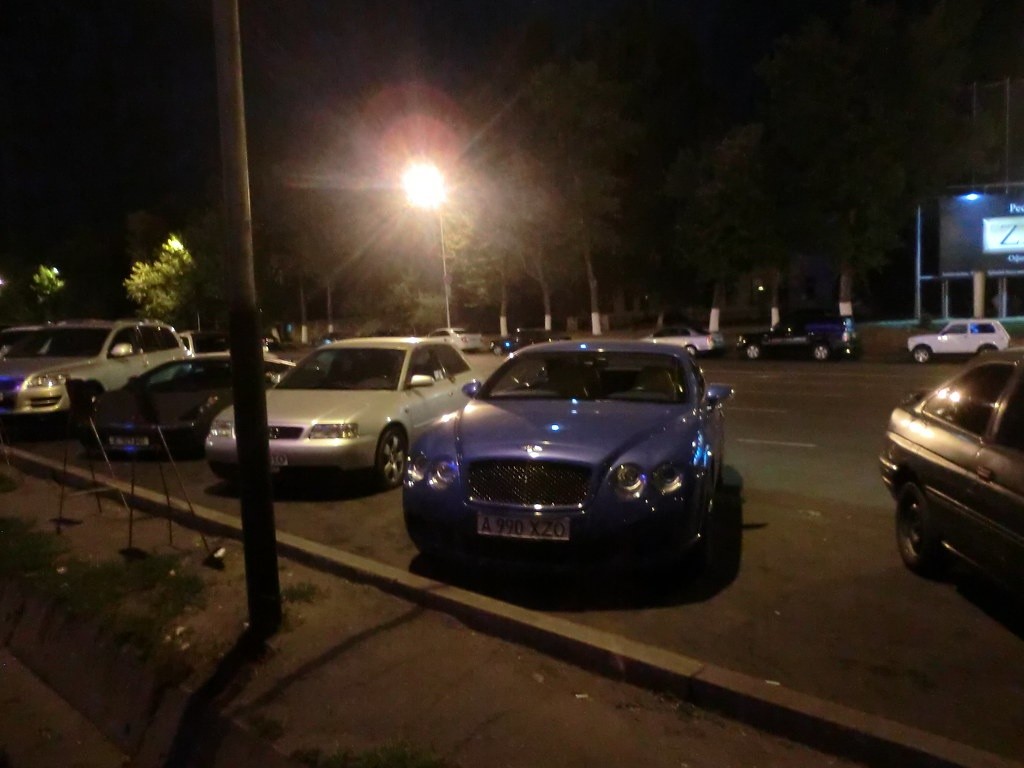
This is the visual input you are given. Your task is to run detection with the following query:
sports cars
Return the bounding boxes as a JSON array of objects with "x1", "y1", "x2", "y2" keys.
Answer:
[
  {"x1": 398, "y1": 338, "x2": 734, "y2": 579},
  {"x1": 82, "y1": 353, "x2": 295, "y2": 457}
]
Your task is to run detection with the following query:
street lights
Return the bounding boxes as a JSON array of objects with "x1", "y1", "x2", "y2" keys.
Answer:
[{"x1": 411, "y1": 161, "x2": 458, "y2": 332}]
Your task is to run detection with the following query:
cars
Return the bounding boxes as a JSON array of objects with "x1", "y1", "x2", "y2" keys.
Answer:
[
  {"x1": 884, "y1": 341, "x2": 1021, "y2": 594},
  {"x1": 737, "y1": 316, "x2": 855, "y2": 364},
  {"x1": 643, "y1": 328, "x2": 724, "y2": 358},
  {"x1": 432, "y1": 326, "x2": 487, "y2": 352},
  {"x1": 905, "y1": 322, "x2": 1009, "y2": 363},
  {"x1": 207, "y1": 335, "x2": 486, "y2": 491}
]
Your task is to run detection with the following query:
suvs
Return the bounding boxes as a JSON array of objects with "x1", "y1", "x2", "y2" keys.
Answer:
[{"x1": 1, "y1": 322, "x2": 184, "y2": 426}]
